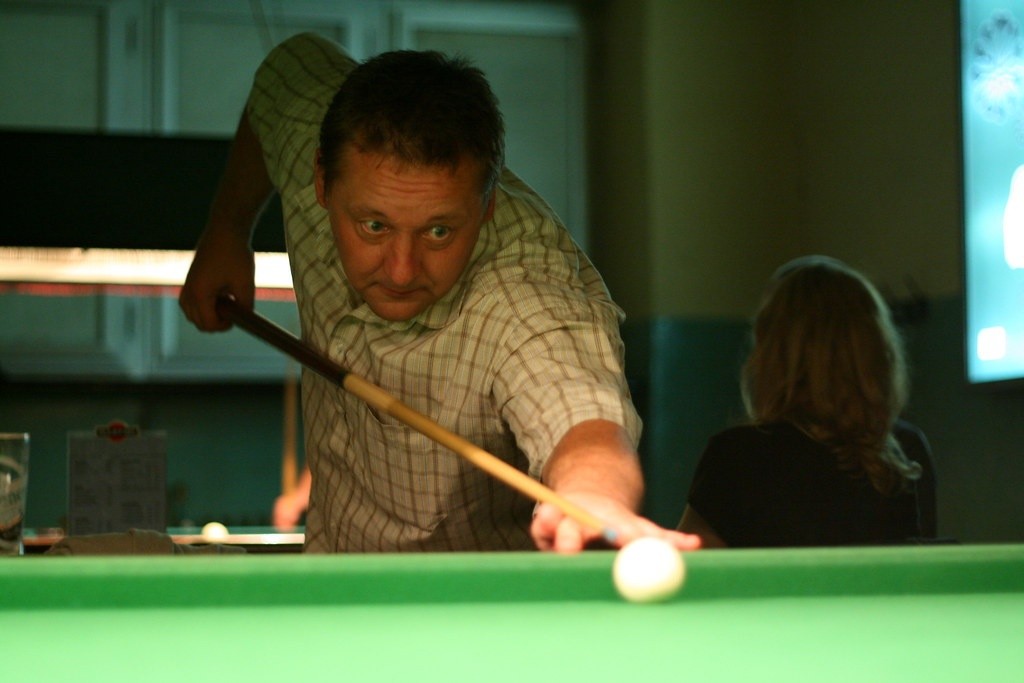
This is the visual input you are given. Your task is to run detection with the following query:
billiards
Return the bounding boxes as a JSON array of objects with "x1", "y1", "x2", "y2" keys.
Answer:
[{"x1": 613, "y1": 537, "x2": 686, "y2": 605}]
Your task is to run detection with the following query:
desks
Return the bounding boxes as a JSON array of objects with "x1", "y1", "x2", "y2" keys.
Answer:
[{"x1": 1, "y1": 525, "x2": 1024, "y2": 683}]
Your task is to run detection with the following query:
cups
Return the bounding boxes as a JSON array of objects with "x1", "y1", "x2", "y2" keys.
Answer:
[{"x1": 0, "y1": 433, "x2": 30, "y2": 555}]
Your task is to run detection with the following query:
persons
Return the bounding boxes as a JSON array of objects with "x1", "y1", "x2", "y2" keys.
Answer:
[
  {"x1": 679, "y1": 257, "x2": 940, "y2": 551},
  {"x1": 180, "y1": 31, "x2": 702, "y2": 557}
]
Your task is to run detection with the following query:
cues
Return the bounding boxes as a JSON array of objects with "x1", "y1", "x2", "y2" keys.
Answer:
[{"x1": 218, "y1": 297, "x2": 619, "y2": 543}]
